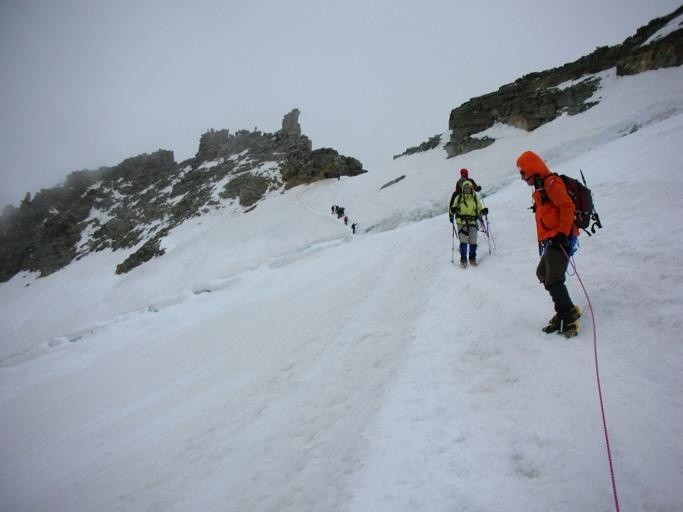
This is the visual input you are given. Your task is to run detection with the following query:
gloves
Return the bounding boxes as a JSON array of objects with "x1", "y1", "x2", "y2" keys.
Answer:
[
  {"x1": 481, "y1": 207, "x2": 489, "y2": 215},
  {"x1": 450, "y1": 206, "x2": 456, "y2": 214},
  {"x1": 554, "y1": 232, "x2": 569, "y2": 248}
]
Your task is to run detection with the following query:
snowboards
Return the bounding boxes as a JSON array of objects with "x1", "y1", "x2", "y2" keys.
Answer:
[{"x1": 564, "y1": 322, "x2": 578, "y2": 338}]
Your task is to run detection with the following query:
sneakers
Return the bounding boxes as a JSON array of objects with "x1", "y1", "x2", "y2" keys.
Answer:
[
  {"x1": 542, "y1": 306, "x2": 580, "y2": 338},
  {"x1": 459, "y1": 257, "x2": 478, "y2": 268}
]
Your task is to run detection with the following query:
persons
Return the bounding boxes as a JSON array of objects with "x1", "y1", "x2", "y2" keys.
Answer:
[
  {"x1": 351, "y1": 222, "x2": 358, "y2": 234},
  {"x1": 455, "y1": 167, "x2": 481, "y2": 195},
  {"x1": 343, "y1": 215, "x2": 348, "y2": 226},
  {"x1": 330, "y1": 204, "x2": 345, "y2": 219},
  {"x1": 514, "y1": 149, "x2": 581, "y2": 337},
  {"x1": 447, "y1": 180, "x2": 488, "y2": 269}
]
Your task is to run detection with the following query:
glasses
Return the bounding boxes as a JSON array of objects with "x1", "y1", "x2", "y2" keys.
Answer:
[{"x1": 519, "y1": 170, "x2": 525, "y2": 175}]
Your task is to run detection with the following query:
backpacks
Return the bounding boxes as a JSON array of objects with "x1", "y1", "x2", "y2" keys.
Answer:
[{"x1": 541, "y1": 172, "x2": 592, "y2": 230}]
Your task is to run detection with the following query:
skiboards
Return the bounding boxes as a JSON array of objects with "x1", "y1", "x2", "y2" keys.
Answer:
[{"x1": 458, "y1": 258, "x2": 478, "y2": 269}]
